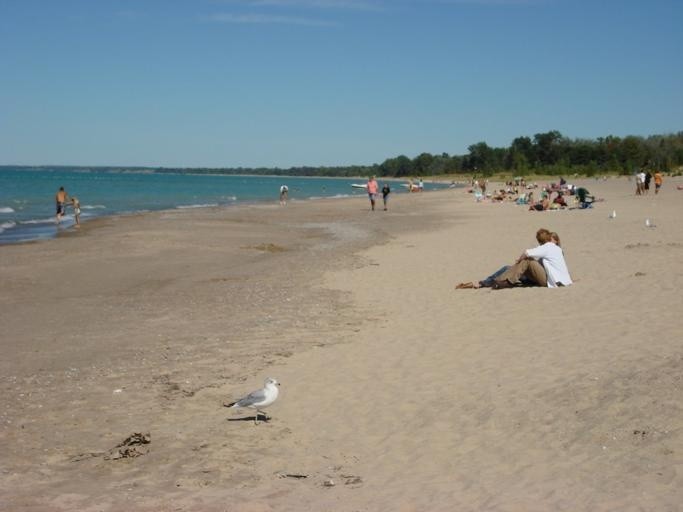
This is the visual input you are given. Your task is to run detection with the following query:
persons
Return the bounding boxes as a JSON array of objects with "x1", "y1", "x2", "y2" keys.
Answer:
[
  {"x1": 408, "y1": 177, "x2": 413, "y2": 193},
  {"x1": 652, "y1": 171, "x2": 662, "y2": 194},
  {"x1": 638, "y1": 168, "x2": 646, "y2": 195},
  {"x1": 493, "y1": 229, "x2": 573, "y2": 288},
  {"x1": 465, "y1": 168, "x2": 596, "y2": 214},
  {"x1": 366, "y1": 174, "x2": 377, "y2": 211},
  {"x1": 278, "y1": 183, "x2": 288, "y2": 207},
  {"x1": 380, "y1": 183, "x2": 390, "y2": 211},
  {"x1": 69, "y1": 197, "x2": 81, "y2": 225},
  {"x1": 454, "y1": 231, "x2": 563, "y2": 288},
  {"x1": 635, "y1": 171, "x2": 643, "y2": 196},
  {"x1": 55, "y1": 187, "x2": 67, "y2": 223},
  {"x1": 418, "y1": 178, "x2": 423, "y2": 192}
]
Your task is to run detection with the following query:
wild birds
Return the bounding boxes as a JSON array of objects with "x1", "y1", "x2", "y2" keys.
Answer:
[
  {"x1": 609, "y1": 210, "x2": 616, "y2": 220},
  {"x1": 222, "y1": 376, "x2": 283, "y2": 427},
  {"x1": 645, "y1": 218, "x2": 657, "y2": 230}
]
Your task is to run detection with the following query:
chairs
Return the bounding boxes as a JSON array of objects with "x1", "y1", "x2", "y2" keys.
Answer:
[{"x1": 574, "y1": 187, "x2": 594, "y2": 208}]
